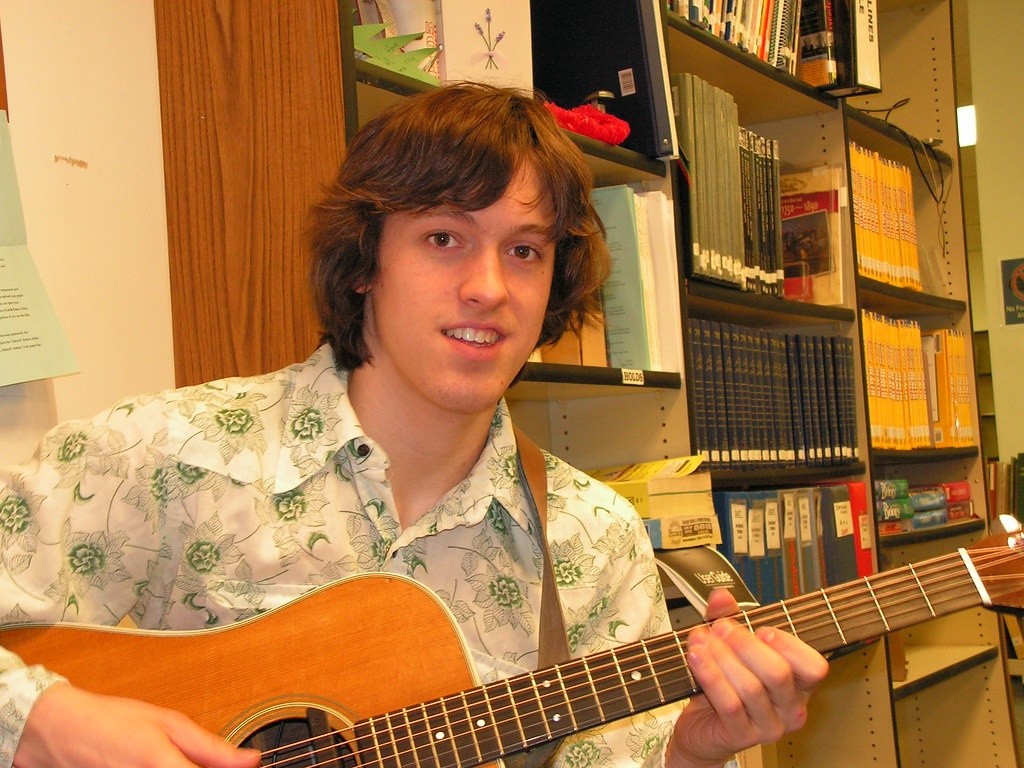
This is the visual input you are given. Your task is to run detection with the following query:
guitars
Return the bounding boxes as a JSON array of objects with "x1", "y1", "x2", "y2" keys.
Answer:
[{"x1": 0, "y1": 513, "x2": 1024, "y2": 768}]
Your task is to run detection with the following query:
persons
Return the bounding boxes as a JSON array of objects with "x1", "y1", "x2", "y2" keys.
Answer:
[{"x1": 2, "y1": 79, "x2": 832, "y2": 768}]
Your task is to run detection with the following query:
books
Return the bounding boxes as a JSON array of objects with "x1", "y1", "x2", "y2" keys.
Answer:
[{"x1": 360, "y1": 0, "x2": 984, "y2": 619}]
